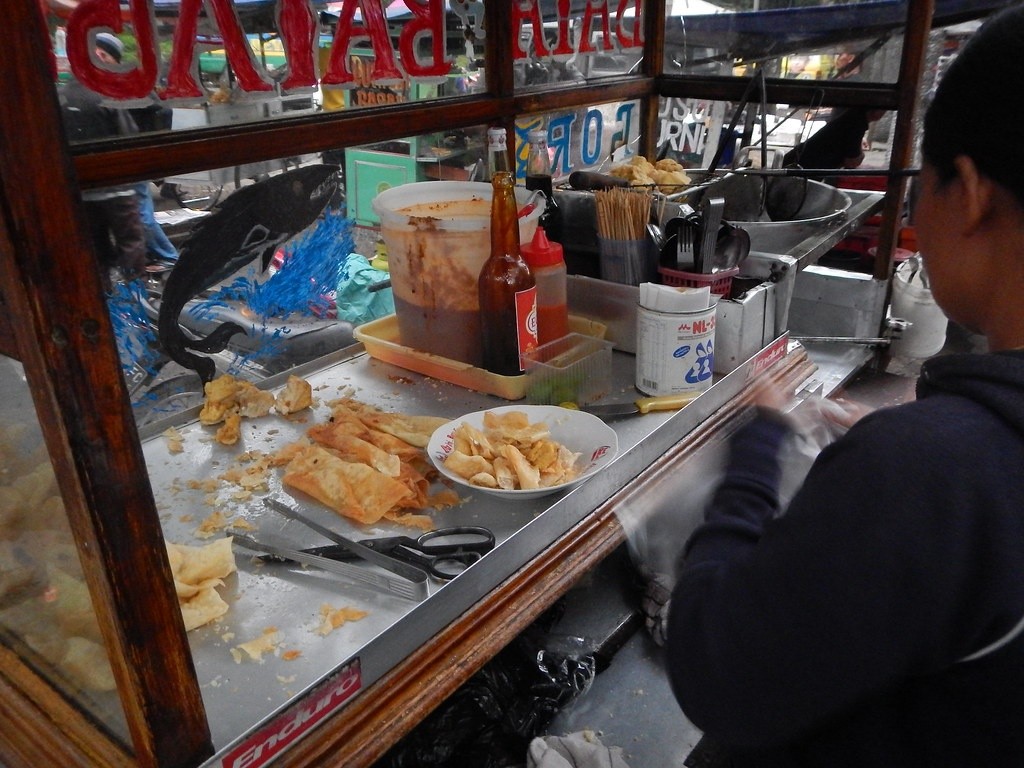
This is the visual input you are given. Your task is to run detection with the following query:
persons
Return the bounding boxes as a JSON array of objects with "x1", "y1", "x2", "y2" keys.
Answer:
[
  {"x1": 780, "y1": 53, "x2": 875, "y2": 150},
  {"x1": 518, "y1": 34, "x2": 584, "y2": 130},
  {"x1": 425, "y1": 53, "x2": 469, "y2": 95},
  {"x1": 663, "y1": 8, "x2": 1024, "y2": 767},
  {"x1": 63, "y1": 32, "x2": 178, "y2": 294}
]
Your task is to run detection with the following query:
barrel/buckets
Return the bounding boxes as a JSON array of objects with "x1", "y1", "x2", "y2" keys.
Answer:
[
  {"x1": 889, "y1": 260, "x2": 950, "y2": 357},
  {"x1": 372, "y1": 181, "x2": 546, "y2": 364}
]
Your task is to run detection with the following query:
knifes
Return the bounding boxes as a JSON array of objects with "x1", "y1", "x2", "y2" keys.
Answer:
[{"x1": 578, "y1": 391, "x2": 703, "y2": 415}]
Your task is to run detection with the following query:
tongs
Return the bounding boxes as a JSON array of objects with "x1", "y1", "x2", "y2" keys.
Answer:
[{"x1": 225, "y1": 496, "x2": 430, "y2": 601}]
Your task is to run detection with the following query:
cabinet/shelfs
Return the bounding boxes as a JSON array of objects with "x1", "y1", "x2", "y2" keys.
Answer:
[
  {"x1": 162, "y1": 92, "x2": 315, "y2": 187},
  {"x1": 346, "y1": 148, "x2": 488, "y2": 228}
]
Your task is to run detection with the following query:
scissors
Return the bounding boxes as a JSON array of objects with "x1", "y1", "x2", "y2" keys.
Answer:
[{"x1": 256, "y1": 527, "x2": 495, "y2": 581}]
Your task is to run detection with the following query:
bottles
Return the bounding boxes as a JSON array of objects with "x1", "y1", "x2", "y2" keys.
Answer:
[
  {"x1": 486, "y1": 126, "x2": 510, "y2": 183},
  {"x1": 523, "y1": 130, "x2": 561, "y2": 242},
  {"x1": 519, "y1": 227, "x2": 571, "y2": 363},
  {"x1": 477, "y1": 172, "x2": 537, "y2": 376}
]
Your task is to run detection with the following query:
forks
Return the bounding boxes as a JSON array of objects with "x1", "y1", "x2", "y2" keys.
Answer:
[{"x1": 676, "y1": 225, "x2": 695, "y2": 273}]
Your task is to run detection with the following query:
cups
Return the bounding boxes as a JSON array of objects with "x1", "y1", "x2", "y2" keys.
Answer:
[
  {"x1": 597, "y1": 233, "x2": 656, "y2": 287},
  {"x1": 636, "y1": 287, "x2": 717, "y2": 398}
]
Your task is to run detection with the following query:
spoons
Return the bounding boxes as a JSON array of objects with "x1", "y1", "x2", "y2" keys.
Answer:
[
  {"x1": 712, "y1": 219, "x2": 751, "y2": 275},
  {"x1": 646, "y1": 224, "x2": 667, "y2": 267},
  {"x1": 664, "y1": 218, "x2": 694, "y2": 265}
]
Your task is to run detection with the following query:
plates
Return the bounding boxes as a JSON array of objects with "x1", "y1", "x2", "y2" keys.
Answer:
[{"x1": 427, "y1": 406, "x2": 618, "y2": 500}]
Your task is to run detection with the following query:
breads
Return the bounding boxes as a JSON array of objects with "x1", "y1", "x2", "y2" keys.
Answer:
[{"x1": 607, "y1": 155, "x2": 689, "y2": 201}]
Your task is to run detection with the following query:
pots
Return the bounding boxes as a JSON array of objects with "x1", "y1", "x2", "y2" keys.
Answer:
[{"x1": 678, "y1": 167, "x2": 852, "y2": 255}]
